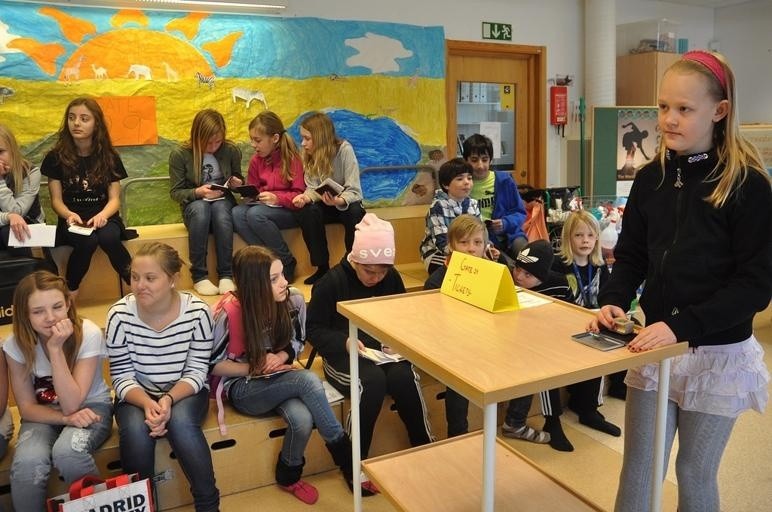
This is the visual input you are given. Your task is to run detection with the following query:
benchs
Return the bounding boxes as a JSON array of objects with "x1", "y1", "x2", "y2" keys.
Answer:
[{"x1": 1, "y1": 201, "x2": 616, "y2": 511}]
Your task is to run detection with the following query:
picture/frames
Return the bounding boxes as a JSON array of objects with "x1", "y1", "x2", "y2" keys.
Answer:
[{"x1": 589, "y1": 104, "x2": 659, "y2": 205}]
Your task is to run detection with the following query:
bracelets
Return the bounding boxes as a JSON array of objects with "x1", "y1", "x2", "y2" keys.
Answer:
[{"x1": 160, "y1": 393, "x2": 174, "y2": 405}]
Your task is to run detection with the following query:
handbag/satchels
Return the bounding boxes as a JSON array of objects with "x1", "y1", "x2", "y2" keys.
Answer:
[{"x1": 0, "y1": 256, "x2": 59, "y2": 326}]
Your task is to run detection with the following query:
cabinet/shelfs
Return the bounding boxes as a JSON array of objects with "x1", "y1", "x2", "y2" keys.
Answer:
[
  {"x1": 616, "y1": 53, "x2": 684, "y2": 108},
  {"x1": 335, "y1": 280, "x2": 690, "y2": 510}
]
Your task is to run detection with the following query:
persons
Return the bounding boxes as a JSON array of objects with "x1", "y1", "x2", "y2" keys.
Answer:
[
  {"x1": 0, "y1": 123, "x2": 59, "y2": 278},
  {"x1": 40, "y1": 97, "x2": 138, "y2": 303},
  {"x1": 421, "y1": 158, "x2": 501, "y2": 274},
  {"x1": 105, "y1": 240, "x2": 220, "y2": 512},
  {"x1": 169, "y1": 108, "x2": 248, "y2": 296},
  {"x1": 513, "y1": 207, "x2": 622, "y2": 451},
  {"x1": 3, "y1": 270, "x2": 113, "y2": 512},
  {"x1": 463, "y1": 134, "x2": 528, "y2": 259},
  {"x1": 209, "y1": 245, "x2": 351, "y2": 504},
  {"x1": 306, "y1": 213, "x2": 440, "y2": 460},
  {"x1": 423, "y1": 214, "x2": 552, "y2": 442},
  {"x1": 293, "y1": 113, "x2": 367, "y2": 284},
  {"x1": 230, "y1": 108, "x2": 305, "y2": 284},
  {"x1": 0, "y1": 344, "x2": 14, "y2": 463},
  {"x1": 585, "y1": 50, "x2": 772, "y2": 512}
]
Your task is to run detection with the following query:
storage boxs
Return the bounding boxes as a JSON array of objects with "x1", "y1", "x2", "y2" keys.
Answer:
[{"x1": 616, "y1": 16, "x2": 682, "y2": 56}]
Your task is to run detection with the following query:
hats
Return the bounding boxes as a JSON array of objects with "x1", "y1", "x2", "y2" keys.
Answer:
[
  {"x1": 513, "y1": 239, "x2": 553, "y2": 283},
  {"x1": 346, "y1": 213, "x2": 396, "y2": 265}
]
